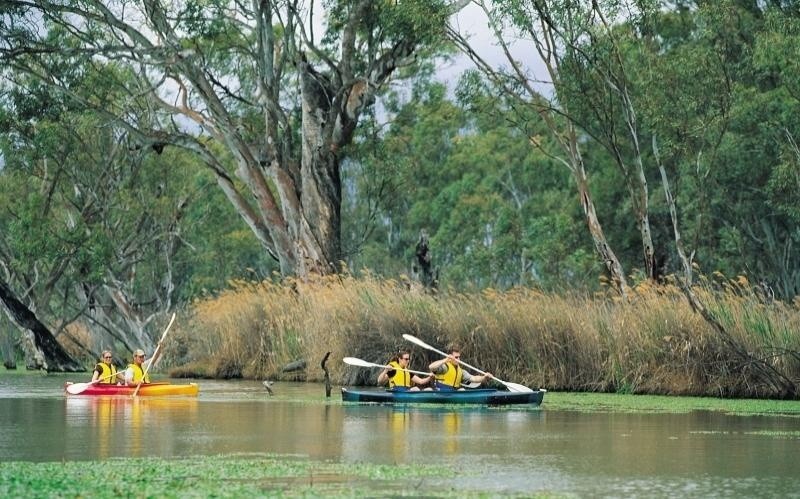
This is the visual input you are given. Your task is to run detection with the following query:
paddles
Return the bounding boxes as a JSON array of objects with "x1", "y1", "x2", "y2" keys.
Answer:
[
  {"x1": 402, "y1": 334, "x2": 532, "y2": 392},
  {"x1": 342, "y1": 357, "x2": 432, "y2": 377},
  {"x1": 67, "y1": 368, "x2": 125, "y2": 394}
]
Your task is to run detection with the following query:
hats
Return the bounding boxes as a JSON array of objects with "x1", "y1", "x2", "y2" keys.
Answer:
[{"x1": 132, "y1": 349, "x2": 146, "y2": 358}]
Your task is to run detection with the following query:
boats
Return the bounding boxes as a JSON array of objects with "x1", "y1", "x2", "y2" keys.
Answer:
[
  {"x1": 62, "y1": 380, "x2": 201, "y2": 395},
  {"x1": 342, "y1": 401, "x2": 546, "y2": 420},
  {"x1": 66, "y1": 394, "x2": 199, "y2": 411},
  {"x1": 340, "y1": 383, "x2": 547, "y2": 407}
]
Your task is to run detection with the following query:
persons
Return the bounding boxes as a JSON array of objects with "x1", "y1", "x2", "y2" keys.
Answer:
[
  {"x1": 92, "y1": 348, "x2": 126, "y2": 384},
  {"x1": 427, "y1": 345, "x2": 495, "y2": 393},
  {"x1": 123, "y1": 341, "x2": 165, "y2": 386},
  {"x1": 376, "y1": 351, "x2": 435, "y2": 393}
]
